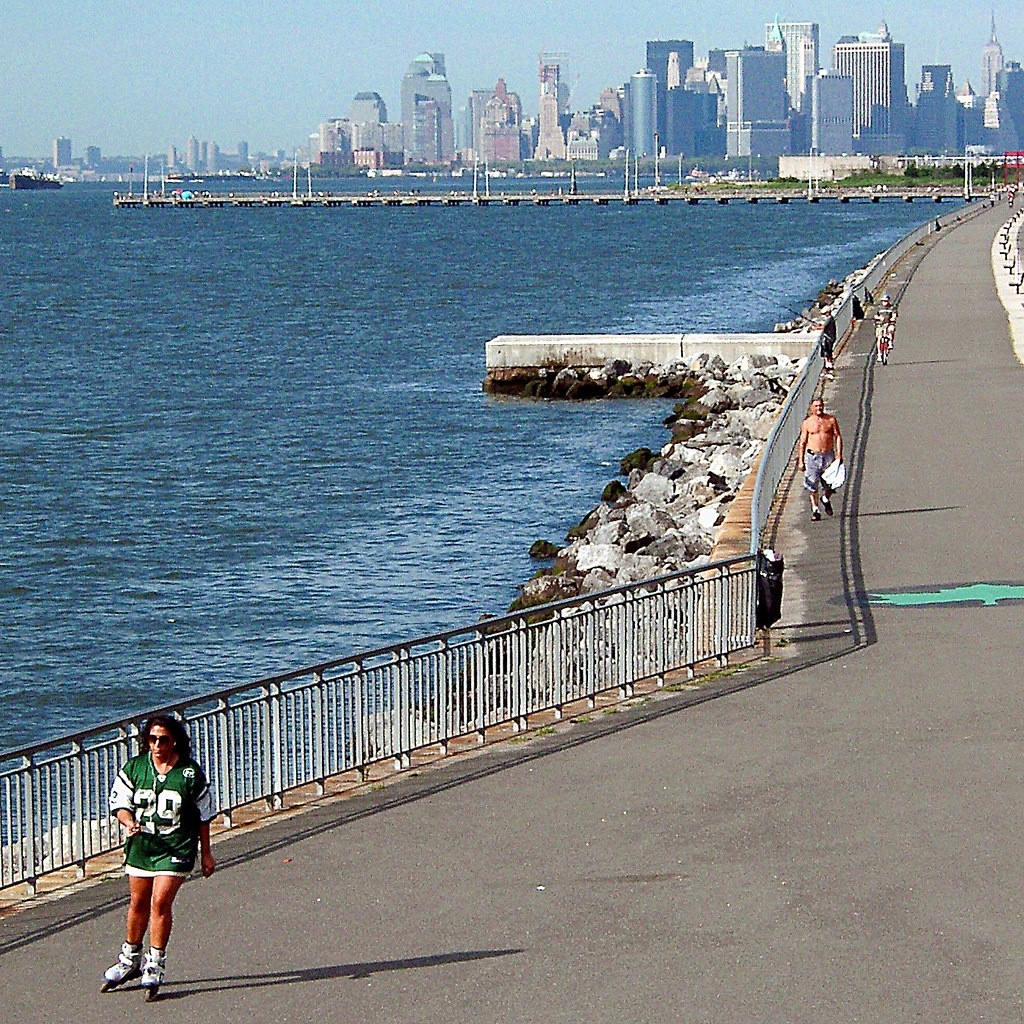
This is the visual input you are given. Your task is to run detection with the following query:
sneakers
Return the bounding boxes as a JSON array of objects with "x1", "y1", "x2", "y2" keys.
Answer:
[
  {"x1": 819, "y1": 494, "x2": 833, "y2": 515},
  {"x1": 811, "y1": 509, "x2": 821, "y2": 521}
]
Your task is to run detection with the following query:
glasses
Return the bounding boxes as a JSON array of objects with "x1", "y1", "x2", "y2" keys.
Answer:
[
  {"x1": 881, "y1": 300, "x2": 887, "y2": 302},
  {"x1": 147, "y1": 734, "x2": 174, "y2": 744}
]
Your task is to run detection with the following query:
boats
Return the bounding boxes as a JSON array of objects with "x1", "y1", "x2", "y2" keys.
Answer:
[{"x1": 9, "y1": 173, "x2": 65, "y2": 191}]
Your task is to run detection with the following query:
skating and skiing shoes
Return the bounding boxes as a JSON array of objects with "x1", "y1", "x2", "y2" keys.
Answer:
[
  {"x1": 99, "y1": 940, "x2": 145, "y2": 993},
  {"x1": 141, "y1": 946, "x2": 168, "y2": 1002}
]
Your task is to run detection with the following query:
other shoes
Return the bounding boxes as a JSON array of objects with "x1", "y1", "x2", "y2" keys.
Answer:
[
  {"x1": 877, "y1": 354, "x2": 882, "y2": 362},
  {"x1": 888, "y1": 342, "x2": 893, "y2": 349}
]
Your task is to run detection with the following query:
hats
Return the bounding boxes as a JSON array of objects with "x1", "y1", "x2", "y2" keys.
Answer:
[
  {"x1": 882, "y1": 294, "x2": 890, "y2": 300},
  {"x1": 820, "y1": 305, "x2": 831, "y2": 313}
]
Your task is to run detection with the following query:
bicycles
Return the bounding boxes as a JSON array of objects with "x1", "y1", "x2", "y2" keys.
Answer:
[{"x1": 870, "y1": 310, "x2": 898, "y2": 365}]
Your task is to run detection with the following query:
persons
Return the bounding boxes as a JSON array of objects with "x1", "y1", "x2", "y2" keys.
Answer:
[
  {"x1": 823, "y1": 310, "x2": 837, "y2": 372},
  {"x1": 799, "y1": 395, "x2": 841, "y2": 522},
  {"x1": 990, "y1": 182, "x2": 1017, "y2": 202},
  {"x1": 258, "y1": 183, "x2": 940, "y2": 198},
  {"x1": 114, "y1": 187, "x2": 234, "y2": 199},
  {"x1": 100, "y1": 714, "x2": 216, "y2": 999},
  {"x1": 872, "y1": 295, "x2": 897, "y2": 361}
]
